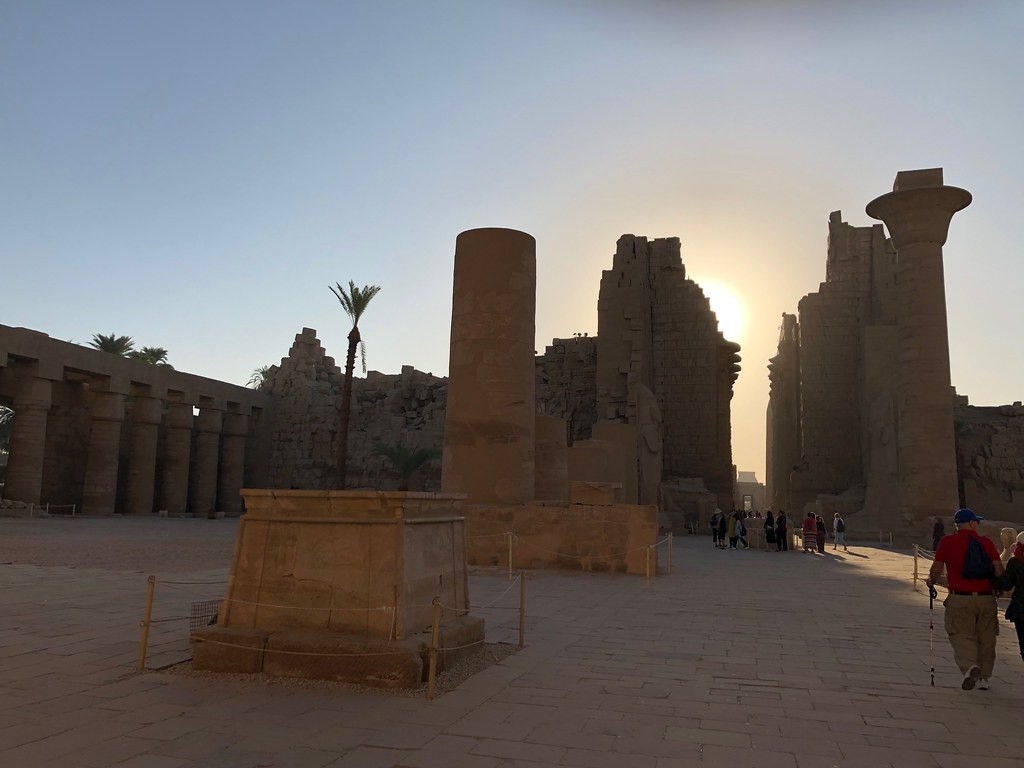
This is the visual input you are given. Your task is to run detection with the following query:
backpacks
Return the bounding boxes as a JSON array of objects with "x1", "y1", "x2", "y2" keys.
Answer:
[
  {"x1": 959, "y1": 537, "x2": 995, "y2": 580},
  {"x1": 710, "y1": 514, "x2": 718, "y2": 526},
  {"x1": 738, "y1": 520, "x2": 747, "y2": 537},
  {"x1": 836, "y1": 519, "x2": 844, "y2": 533}
]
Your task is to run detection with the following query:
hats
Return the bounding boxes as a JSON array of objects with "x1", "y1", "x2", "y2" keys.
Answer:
[
  {"x1": 714, "y1": 508, "x2": 722, "y2": 513},
  {"x1": 1016, "y1": 531, "x2": 1024, "y2": 545},
  {"x1": 954, "y1": 508, "x2": 984, "y2": 523}
]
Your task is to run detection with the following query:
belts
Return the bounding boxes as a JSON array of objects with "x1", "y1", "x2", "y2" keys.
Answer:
[{"x1": 949, "y1": 590, "x2": 993, "y2": 596}]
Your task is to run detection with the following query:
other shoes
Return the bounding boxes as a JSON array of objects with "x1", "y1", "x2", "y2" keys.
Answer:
[
  {"x1": 811, "y1": 551, "x2": 814, "y2": 553},
  {"x1": 961, "y1": 665, "x2": 981, "y2": 690},
  {"x1": 715, "y1": 545, "x2": 718, "y2": 547},
  {"x1": 730, "y1": 547, "x2": 736, "y2": 550},
  {"x1": 844, "y1": 548, "x2": 847, "y2": 551},
  {"x1": 977, "y1": 678, "x2": 989, "y2": 689},
  {"x1": 833, "y1": 548, "x2": 836, "y2": 550},
  {"x1": 805, "y1": 550, "x2": 808, "y2": 551},
  {"x1": 775, "y1": 550, "x2": 779, "y2": 552},
  {"x1": 720, "y1": 545, "x2": 727, "y2": 547},
  {"x1": 764, "y1": 550, "x2": 770, "y2": 552},
  {"x1": 744, "y1": 547, "x2": 749, "y2": 550}
]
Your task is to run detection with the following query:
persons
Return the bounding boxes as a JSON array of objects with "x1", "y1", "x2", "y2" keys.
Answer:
[
  {"x1": 802, "y1": 512, "x2": 826, "y2": 553},
  {"x1": 932, "y1": 517, "x2": 945, "y2": 551},
  {"x1": 727, "y1": 511, "x2": 736, "y2": 548},
  {"x1": 729, "y1": 508, "x2": 762, "y2": 519},
  {"x1": 995, "y1": 531, "x2": 1024, "y2": 662},
  {"x1": 711, "y1": 508, "x2": 726, "y2": 549},
  {"x1": 730, "y1": 514, "x2": 749, "y2": 550},
  {"x1": 775, "y1": 510, "x2": 788, "y2": 552},
  {"x1": 926, "y1": 509, "x2": 1005, "y2": 690},
  {"x1": 833, "y1": 513, "x2": 847, "y2": 551},
  {"x1": 763, "y1": 511, "x2": 776, "y2": 551}
]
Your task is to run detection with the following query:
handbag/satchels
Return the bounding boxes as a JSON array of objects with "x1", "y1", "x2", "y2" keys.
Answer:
[{"x1": 767, "y1": 526, "x2": 772, "y2": 530}]
